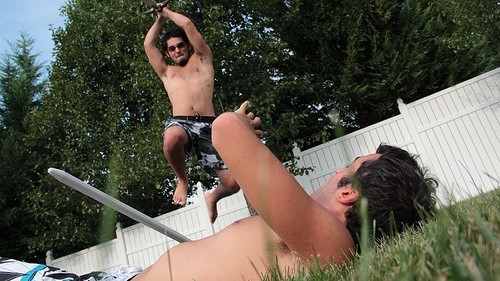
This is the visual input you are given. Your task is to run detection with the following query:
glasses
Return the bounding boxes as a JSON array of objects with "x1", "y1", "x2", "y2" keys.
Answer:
[{"x1": 166, "y1": 41, "x2": 187, "y2": 51}]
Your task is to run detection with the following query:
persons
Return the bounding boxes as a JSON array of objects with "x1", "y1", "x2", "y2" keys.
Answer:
[
  {"x1": 0, "y1": 100, "x2": 439, "y2": 281},
  {"x1": 143, "y1": 0, "x2": 240, "y2": 224}
]
